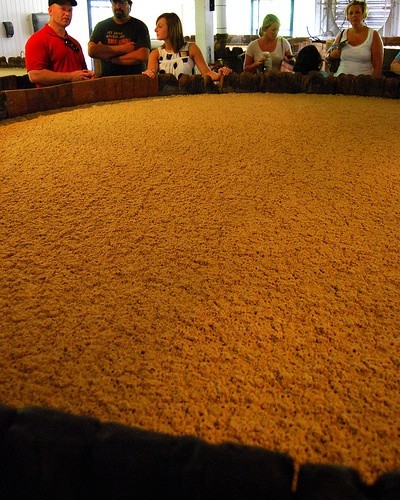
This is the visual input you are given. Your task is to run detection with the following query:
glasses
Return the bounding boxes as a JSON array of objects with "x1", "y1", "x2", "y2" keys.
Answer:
[
  {"x1": 111, "y1": 1, "x2": 128, "y2": 6},
  {"x1": 65, "y1": 39, "x2": 80, "y2": 53}
]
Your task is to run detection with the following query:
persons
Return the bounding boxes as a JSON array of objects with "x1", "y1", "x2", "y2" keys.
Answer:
[
  {"x1": 25, "y1": 0, "x2": 94, "y2": 88},
  {"x1": 390, "y1": 51, "x2": 400, "y2": 75},
  {"x1": 244, "y1": 14, "x2": 296, "y2": 74},
  {"x1": 325, "y1": 0, "x2": 384, "y2": 77},
  {"x1": 88, "y1": 0, "x2": 152, "y2": 78},
  {"x1": 142, "y1": 13, "x2": 233, "y2": 81},
  {"x1": 292, "y1": 43, "x2": 325, "y2": 77}
]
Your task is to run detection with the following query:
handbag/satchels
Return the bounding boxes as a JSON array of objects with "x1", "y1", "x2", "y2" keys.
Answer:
[
  {"x1": 329, "y1": 29, "x2": 344, "y2": 73},
  {"x1": 280, "y1": 37, "x2": 294, "y2": 73}
]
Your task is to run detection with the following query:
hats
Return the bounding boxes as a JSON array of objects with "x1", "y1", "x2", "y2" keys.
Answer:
[{"x1": 48, "y1": 0, "x2": 78, "y2": 6}]
